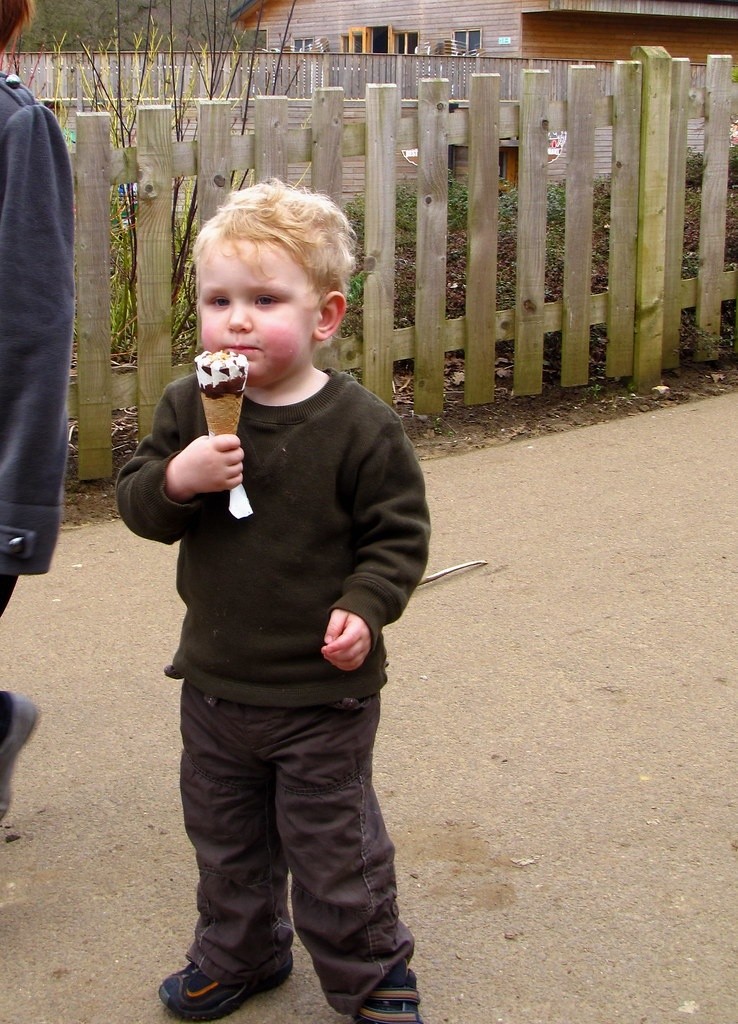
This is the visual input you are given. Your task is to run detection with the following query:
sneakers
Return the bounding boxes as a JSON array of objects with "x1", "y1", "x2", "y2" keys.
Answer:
[
  {"x1": 0, "y1": 693, "x2": 36, "y2": 820},
  {"x1": 158, "y1": 950, "x2": 293, "y2": 1021},
  {"x1": 353, "y1": 958, "x2": 423, "y2": 1024}
]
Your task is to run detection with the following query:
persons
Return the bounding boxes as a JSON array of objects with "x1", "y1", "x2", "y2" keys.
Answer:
[
  {"x1": 115, "y1": 179, "x2": 432, "y2": 1022},
  {"x1": 0, "y1": 0, "x2": 75, "y2": 825}
]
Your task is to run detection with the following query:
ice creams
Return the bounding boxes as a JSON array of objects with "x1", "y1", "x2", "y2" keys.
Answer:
[{"x1": 193, "y1": 349, "x2": 248, "y2": 435}]
